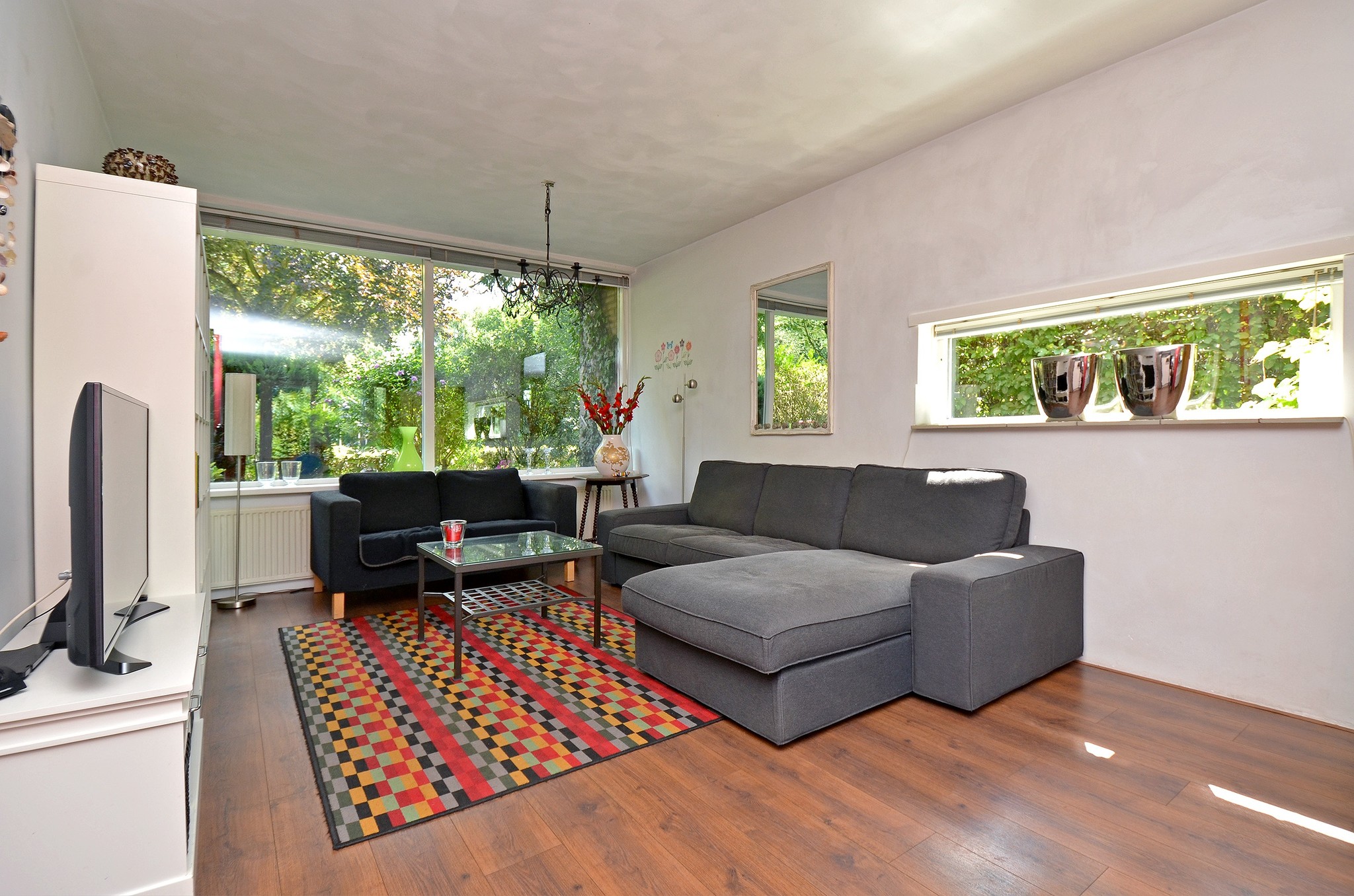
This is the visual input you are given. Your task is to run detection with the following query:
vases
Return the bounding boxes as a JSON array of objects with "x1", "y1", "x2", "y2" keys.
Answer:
[
  {"x1": 593, "y1": 435, "x2": 631, "y2": 475},
  {"x1": 393, "y1": 426, "x2": 424, "y2": 471}
]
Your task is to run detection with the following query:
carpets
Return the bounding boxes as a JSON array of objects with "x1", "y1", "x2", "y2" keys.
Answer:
[{"x1": 277, "y1": 585, "x2": 726, "y2": 852}]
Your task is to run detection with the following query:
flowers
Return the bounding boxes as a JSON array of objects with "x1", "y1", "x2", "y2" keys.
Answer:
[{"x1": 565, "y1": 375, "x2": 653, "y2": 436}]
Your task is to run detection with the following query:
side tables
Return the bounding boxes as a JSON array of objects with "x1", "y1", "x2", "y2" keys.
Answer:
[{"x1": 574, "y1": 473, "x2": 649, "y2": 540}]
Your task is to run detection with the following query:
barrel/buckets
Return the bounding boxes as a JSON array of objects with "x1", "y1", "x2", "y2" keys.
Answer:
[
  {"x1": 1112, "y1": 343, "x2": 1197, "y2": 419},
  {"x1": 1030, "y1": 353, "x2": 1099, "y2": 423}
]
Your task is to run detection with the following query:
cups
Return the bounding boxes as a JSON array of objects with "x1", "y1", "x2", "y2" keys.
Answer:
[{"x1": 440, "y1": 519, "x2": 466, "y2": 549}]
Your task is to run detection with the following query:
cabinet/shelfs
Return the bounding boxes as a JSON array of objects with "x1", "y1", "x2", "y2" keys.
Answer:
[{"x1": 0, "y1": 162, "x2": 212, "y2": 896}]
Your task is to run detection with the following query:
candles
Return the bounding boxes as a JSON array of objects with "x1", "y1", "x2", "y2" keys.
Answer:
[{"x1": 441, "y1": 520, "x2": 467, "y2": 548}]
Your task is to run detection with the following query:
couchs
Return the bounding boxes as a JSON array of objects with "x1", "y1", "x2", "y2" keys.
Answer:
[{"x1": 594, "y1": 458, "x2": 1085, "y2": 747}]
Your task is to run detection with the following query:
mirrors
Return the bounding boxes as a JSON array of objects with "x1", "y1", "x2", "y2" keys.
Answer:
[{"x1": 748, "y1": 260, "x2": 836, "y2": 435}]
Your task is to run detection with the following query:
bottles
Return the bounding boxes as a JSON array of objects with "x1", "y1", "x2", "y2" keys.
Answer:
[{"x1": 394, "y1": 426, "x2": 423, "y2": 473}]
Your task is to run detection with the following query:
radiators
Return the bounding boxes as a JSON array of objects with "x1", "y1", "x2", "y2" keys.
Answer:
[
  {"x1": 211, "y1": 504, "x2": 312, "y2": 590},
  {"x1": 576, "y1": 486, "x2": 612, "y2": 540}
]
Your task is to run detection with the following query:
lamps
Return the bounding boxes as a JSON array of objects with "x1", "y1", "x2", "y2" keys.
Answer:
[
  {"x1": 672, "y1": 372, "x2": 698, "y2": 503},
  {"x1": 216, "y1": 371, "x2": 259, "y2": 610},
  {"x1": 489, "y1": 178, "x2": 605, "y2": 328}
]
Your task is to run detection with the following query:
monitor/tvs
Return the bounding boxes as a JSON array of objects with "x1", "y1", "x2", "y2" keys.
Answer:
[{"x1": 64, "y1": 382, "x2": 169, "y2": 675}]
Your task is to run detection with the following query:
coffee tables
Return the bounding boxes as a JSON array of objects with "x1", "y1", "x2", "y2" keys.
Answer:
[{"x1": 415, "y1": 530, "x2": 604, "y2": 684}]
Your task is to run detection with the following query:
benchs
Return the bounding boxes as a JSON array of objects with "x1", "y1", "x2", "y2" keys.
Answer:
[{"x1": 310, "y1": 469, "x2": 577, "y2": 620}]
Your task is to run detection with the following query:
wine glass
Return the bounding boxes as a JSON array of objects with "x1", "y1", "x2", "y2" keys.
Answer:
[
  {"x1": 256, "y1": 461, "x2": 278, "y2": 488},
  {"x1": 280, "y1": 461, "x2": 302, "y2": 488}
]
